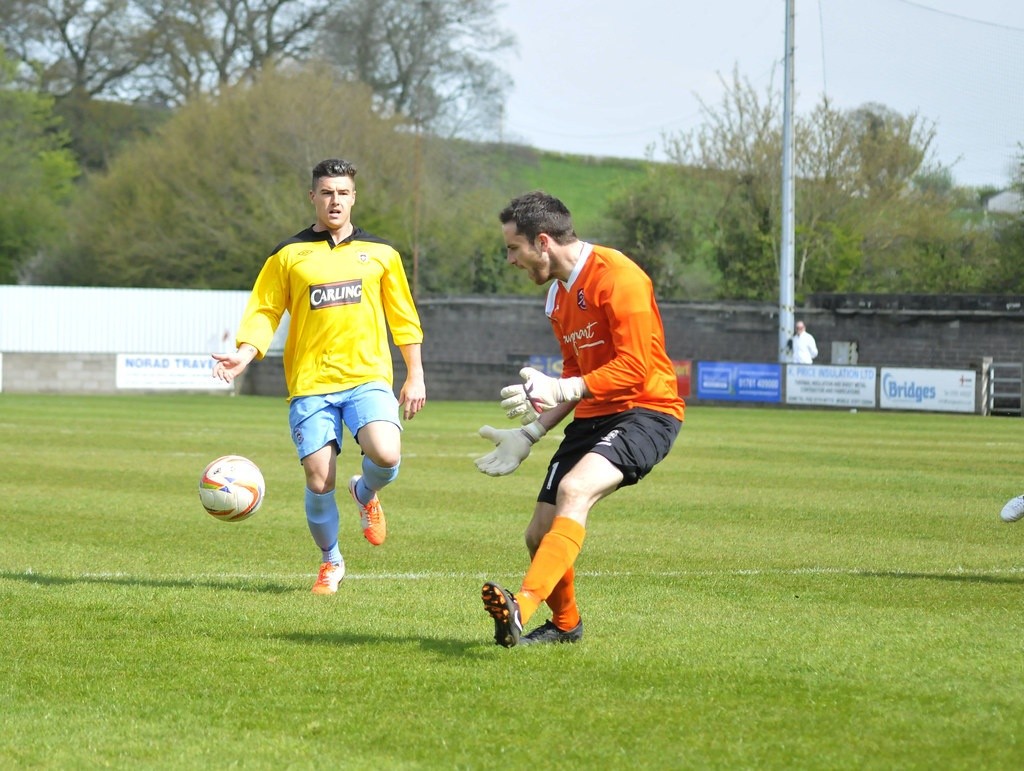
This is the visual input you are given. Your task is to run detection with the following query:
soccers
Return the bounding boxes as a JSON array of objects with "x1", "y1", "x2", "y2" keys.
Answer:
[{"x1": 198, "y1": 454, "x2": 266, "y2": 523}]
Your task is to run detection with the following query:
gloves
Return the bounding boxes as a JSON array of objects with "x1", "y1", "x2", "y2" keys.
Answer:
[
  {"x1": 501, "y1": 367, "x2": 583, "y2": 426},
  {"x1": 474, "y1": 420, "x2": 546, "y2": 477}
]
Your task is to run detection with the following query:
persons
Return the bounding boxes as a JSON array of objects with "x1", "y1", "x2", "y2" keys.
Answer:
[
  {"x1": 792, "y1": 320, "x2": 818, "y2": 364},
  {"x1": 473, "y1": 191, "x2": 687, "y2": 649},
  {"x1": 211, "y1": 159, "x2": 427, "y2": 595},
  {"x1": 1000, "y1": 495, "x2": 1024, "y2": 522}
]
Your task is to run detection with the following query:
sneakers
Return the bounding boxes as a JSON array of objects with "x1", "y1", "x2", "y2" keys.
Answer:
[
  {"x1": 521, "y1": 616, "x2": 583, "y2": 646},
  {"x1": 312, "y1": 557, "x2": 344, "y2": 595},
  {"x1": 347, "y1": 475, "x2": 386, "y2": 545},
  {"x1": 482, "y1": 581, "x2": 524, "y2": 648}
]
